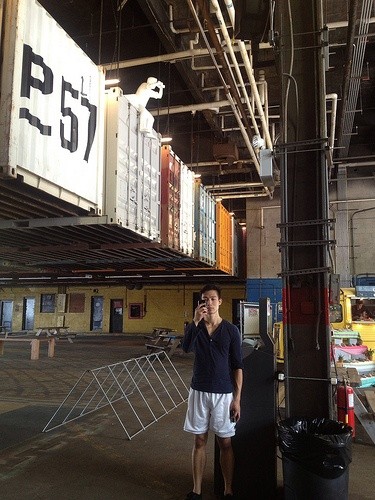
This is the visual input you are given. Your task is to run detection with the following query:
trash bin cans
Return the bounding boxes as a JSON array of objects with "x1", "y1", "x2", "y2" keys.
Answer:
[{"x1": 276, "y1": 414, "x2": 353, "y2": 500}]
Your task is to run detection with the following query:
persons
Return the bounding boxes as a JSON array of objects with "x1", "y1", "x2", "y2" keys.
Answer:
[
  {"x1": 351, "y1": 299, "x2": 375, "y2": 321},
  {"x1": 182, "y1": 285, "x2": 243, "y2": 500}
]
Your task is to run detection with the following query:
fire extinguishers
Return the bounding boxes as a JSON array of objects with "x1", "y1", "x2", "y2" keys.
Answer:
[{"x1": 337, "y1": 377, "x2": 355, "y2": 438}]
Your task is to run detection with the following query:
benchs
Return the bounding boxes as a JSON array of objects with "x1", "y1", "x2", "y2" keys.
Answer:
[
  {"x1": 0, "y1": 326, "x2": 9, "y2": 333},
  {"x1": 48, "y1": 335, "x2": 76, "y2": 345},
  {"x1": 1, "y1": 330, "x2": 32, "y2": 338},
  {"x1": 144, "y1": 326, "x2": 184, "y2": 361}
]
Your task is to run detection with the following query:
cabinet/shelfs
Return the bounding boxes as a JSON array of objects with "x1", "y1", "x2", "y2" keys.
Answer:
[{"x1": 317, "y1": 324, "x2": 375, "y2": 445}]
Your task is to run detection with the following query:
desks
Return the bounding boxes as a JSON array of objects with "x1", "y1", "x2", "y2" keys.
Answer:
[
  {"x1": 29, "y1": 326, "x2": 76, "y2": 345},
  {"x1": 0, "y1": 335, "x2": 57, "y2": 360}
]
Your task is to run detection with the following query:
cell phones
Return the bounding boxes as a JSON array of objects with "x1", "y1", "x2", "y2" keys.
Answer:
[{"x1": 198, "y1": 300, "x2": 205, "y2": 308}]
